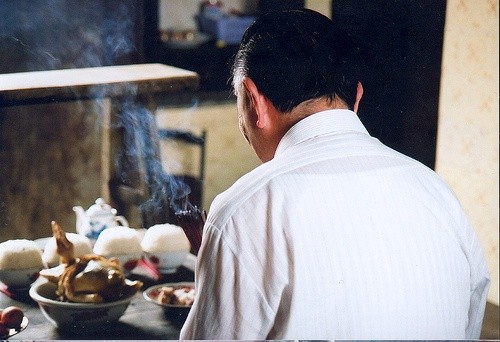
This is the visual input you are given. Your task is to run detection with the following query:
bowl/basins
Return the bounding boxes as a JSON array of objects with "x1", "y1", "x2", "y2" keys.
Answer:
[
  {"x1": 103, "y1": 253, "x2": 142, "y2": 278},
  {"x1": 0, "y1": 266, "x2": 42, "y2": 291},
  {"x1": 28, "y1": 280, "x2": 139, "y2": 333},
  {"x1": 41, "y1": 260, "x2": 66, "y2": 270},
  {"x1": 143, "y1": 251, "x2": 190, "y2": 275}
]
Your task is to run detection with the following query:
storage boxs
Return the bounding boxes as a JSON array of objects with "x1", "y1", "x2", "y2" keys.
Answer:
[{"x1": 194, "y1": 14, "x2": 256, "y2": 44}]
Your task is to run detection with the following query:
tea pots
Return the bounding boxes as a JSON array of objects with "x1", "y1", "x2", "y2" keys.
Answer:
[{"x1": 72, "y1": 198, "x2": 129, "y2": 249}]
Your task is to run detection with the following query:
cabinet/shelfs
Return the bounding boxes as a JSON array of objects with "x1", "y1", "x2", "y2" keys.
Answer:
[{"x1": 156, "y1": 44, "x2": 240, "y2": 105}]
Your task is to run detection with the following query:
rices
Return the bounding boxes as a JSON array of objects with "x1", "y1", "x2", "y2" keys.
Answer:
[
  {"x1": 93, "y1": 226, "x2": 142, "y2": 276},
  {"x1": 0, "y1": 239, "x2": 43, "y2": 292},
  {"x1": 141, "y1": 224, "x2": 192, "y2": 274},
  {"x1": 43, "y1": 231, "x2": 92, "y2": 268}
]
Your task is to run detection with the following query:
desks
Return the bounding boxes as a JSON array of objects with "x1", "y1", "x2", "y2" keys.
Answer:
[{"x1": 0, "y1": 252, "x2": 196, "y2": 340}]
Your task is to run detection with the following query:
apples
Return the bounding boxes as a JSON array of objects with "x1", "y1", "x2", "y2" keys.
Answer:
[{"x1": 2, "y1": 306, "x2": 24, "y2": 329}]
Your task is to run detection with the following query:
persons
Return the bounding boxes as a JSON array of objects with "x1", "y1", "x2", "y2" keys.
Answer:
[{"x1": 179, "y1": 8, "x2": 491, "y2": 340}]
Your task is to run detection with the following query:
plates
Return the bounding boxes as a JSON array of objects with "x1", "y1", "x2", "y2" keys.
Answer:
[
  {"x1": 0, "y1": 309, "x2": 28, "y2": 340},
  {"x1": 142, "y1": 270, "x2": 193, "y2": 312}
]
